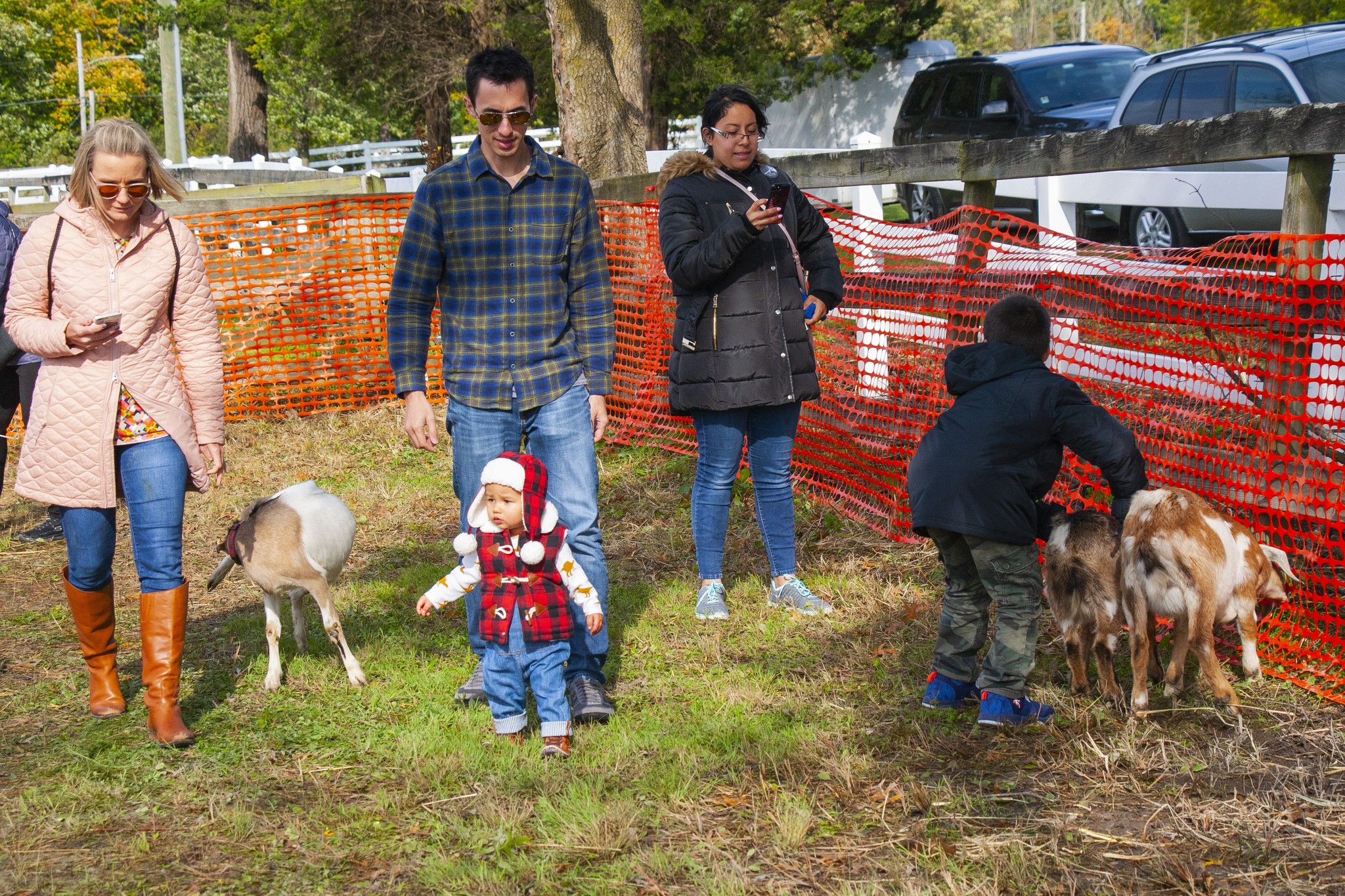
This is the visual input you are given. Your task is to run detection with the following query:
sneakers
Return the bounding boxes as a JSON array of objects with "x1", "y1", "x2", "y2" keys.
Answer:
[
  {"x1": 977, "y1": 690, "x2": 1055, "y2": 727},
  {"x1": 541, "y1": 728, "x2": 571, "y2": 758},
  {"x1": 922, "y1": 668, "x2": 981, "y2": 710},
  {"x1": 482, "y1": 728, "x2": 524, "y2": 750},
  {"x1": 768, "y1": 574, "x2": 833, "y2": 616},
  {"x1": 454, "y1": 661, "x2": 487, "y2": 702},
  {"x1": 694, "y1": 579, "x2": 729, "y2": 620},
  {"x1": 568, "y1": 679, "x2": 614, "y2": 723},
  {"x1": 18, "y1": 516, "x2": 65, "y2": 544}
]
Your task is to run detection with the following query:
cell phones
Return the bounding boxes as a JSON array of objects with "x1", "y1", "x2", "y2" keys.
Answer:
[
  {"x1": 764, "y1": 183, "x2": 791, "y2": 224},
  {"x1": 94, "y1": 311, "x2": 122, "y2": 326}
]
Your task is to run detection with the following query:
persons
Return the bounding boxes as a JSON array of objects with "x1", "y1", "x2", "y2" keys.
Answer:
[
  {"x1": 2, "y1": 112, "x2": 234, "y2": 745},
  {"x1": 652, "y1": 87, "x2": 845, "y2": 620},
  {"x1": 379, "y1": 48, "x2": 620, "y2": 727},
  {"x1": 0, "y1": 200, "x2": 67, "y2": 545},
  {"x1": 419, "y1": 446, "x2": 602, "y2": 759},
  {"x1": 904, "y1": 295, "x2": 1148, "y2": 731}
]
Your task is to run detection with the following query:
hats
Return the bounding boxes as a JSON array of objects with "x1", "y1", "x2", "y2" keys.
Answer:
[{"x1": 453, "y1": 452, "x2": 548, "y2": 565}]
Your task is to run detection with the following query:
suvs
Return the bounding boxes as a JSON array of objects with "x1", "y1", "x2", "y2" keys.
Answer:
[
  {"x1": 1098, "y1": 19, "x2": 1345, "y2": 263},
  {"x1": 893, "y1": 41, "x2": 1153, "y2": 251}
]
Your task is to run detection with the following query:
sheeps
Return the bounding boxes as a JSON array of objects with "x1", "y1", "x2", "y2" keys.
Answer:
[
  {"x1": 206, "y1": 478, "x2": 366, "y2": 691},
  {"x1": 1042, "y1": 485, "x2": 1301, "y2": 724}
]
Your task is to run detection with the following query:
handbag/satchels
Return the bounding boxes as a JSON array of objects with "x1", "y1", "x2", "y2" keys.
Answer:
[{"x1": 0, "y1": 316, "x2": 20, "y2": 371}]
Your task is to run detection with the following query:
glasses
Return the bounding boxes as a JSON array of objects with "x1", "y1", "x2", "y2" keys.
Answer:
[
  {"x1": 89, "y1": 170, "x2": 151, "y2": 199},
  {"x1": 471, "y1": 98, "x2": 534, "y2": 127},
  {"x1": 710, "y1": 126, "x2": 764, "y2": 142}
]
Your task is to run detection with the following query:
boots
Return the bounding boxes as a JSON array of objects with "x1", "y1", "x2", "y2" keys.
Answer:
[
  {"x1": 59, "y1": 565, "x2": 127, "y2": 720},
  {"x1": 139, "y1": 577, "x2": 196, "y2": 748}
]
